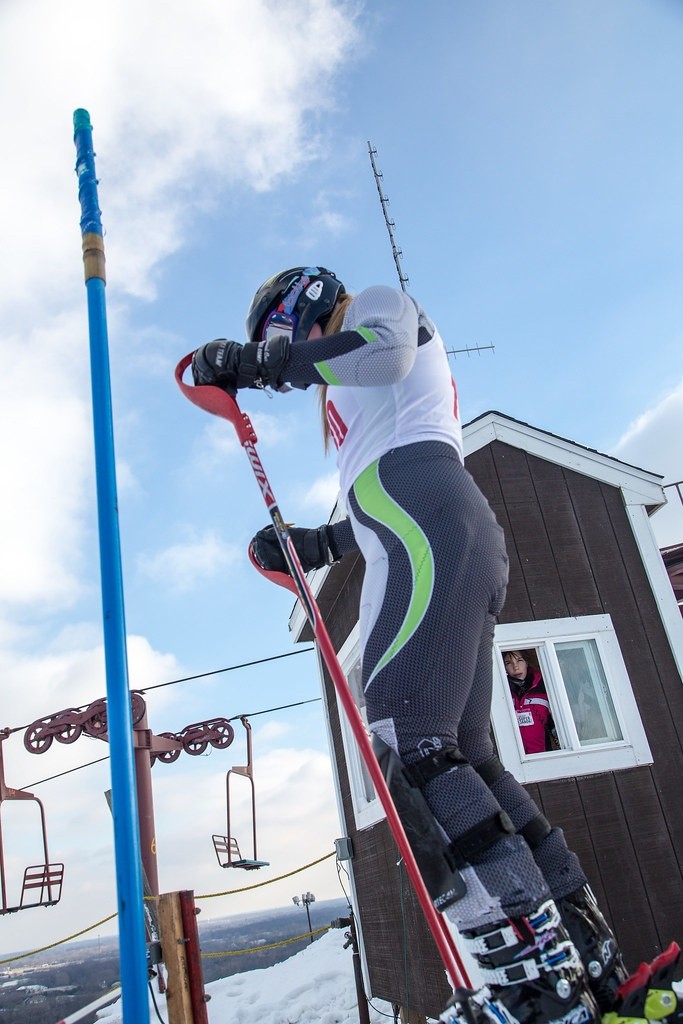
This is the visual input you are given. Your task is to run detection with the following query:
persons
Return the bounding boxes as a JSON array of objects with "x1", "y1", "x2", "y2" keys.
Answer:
[
  {"x1": 191, "y1": 267, "x2": 634, "y2": 1024},
  {"x1": 501, "y1": 650, "x2": 561, "y2": 754}
]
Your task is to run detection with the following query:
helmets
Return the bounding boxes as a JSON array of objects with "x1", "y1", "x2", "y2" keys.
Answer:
[{"x1": 245, "y1": 267, "x2": 346, "y2": 342}]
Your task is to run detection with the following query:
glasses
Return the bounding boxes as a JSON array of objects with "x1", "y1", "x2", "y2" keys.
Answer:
[{"x1": 263, "y1": 267, "x2": 336, "y2": 343}]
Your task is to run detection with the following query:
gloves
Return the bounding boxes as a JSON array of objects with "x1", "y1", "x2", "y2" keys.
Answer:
[
  {"x1": 251, "y1": 524, "x2": 330, "y2": 576},
  {"x1": 191, "y1": 335, "x2": 292, "y2": 397}
]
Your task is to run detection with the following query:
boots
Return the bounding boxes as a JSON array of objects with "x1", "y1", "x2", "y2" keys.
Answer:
[
  {"x1": 554, "y1": 880, "x2": 629, "y2": 1015},
  {"x1": 432, "y1": 899, "x2": 603, "y2": 1024}
]
